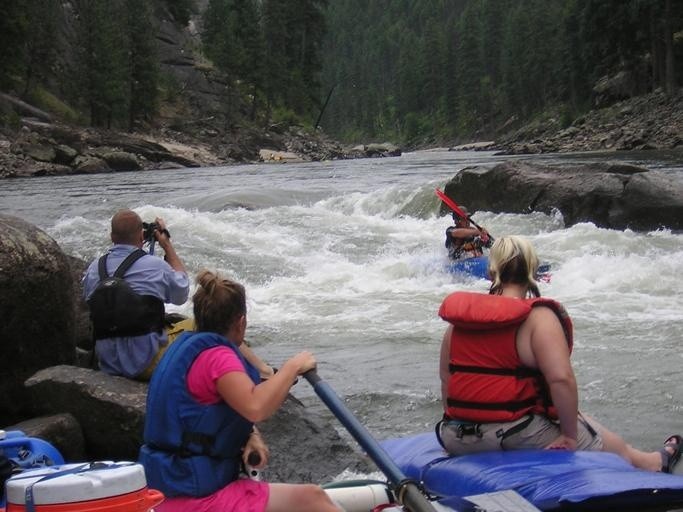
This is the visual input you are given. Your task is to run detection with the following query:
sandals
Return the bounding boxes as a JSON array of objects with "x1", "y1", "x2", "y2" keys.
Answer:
[{"x1": 657, "y1": 434, "x2": 683, "y2": 475}]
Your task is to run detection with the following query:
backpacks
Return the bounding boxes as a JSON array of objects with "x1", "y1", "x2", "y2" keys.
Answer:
[{"x1": 86, "y1": 249, "x2": 148, "y2": 338}]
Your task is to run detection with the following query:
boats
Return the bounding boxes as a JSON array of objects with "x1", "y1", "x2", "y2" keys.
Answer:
[{"x1": 445, "y1": 256, "x2": 551, "y2": 279}]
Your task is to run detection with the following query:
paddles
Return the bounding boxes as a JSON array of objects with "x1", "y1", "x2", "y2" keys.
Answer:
[{"x1": 435, "y1": 188, "x2": 496, "y2": 241}]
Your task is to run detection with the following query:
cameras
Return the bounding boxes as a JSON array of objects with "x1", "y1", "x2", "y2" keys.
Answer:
[{"x1": 143, "y1": 221, "x2": 162, "y2": 242}]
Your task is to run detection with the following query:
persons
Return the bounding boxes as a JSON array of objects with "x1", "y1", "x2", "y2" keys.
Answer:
[
  {"x1": 80, "y1": 211, "x2": 301, "y2": 389},
  {"x1": 442, "y1": 202, "x2": 497, "y2": 261},
  {"x1": 437, "y1": 233, "x2": 682, "y2": 473},
  {"x1": 139, "y1": 272, "x2": 342, "y2": 512}
]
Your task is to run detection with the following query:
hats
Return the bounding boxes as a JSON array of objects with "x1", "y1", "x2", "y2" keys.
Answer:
[{"x1": 452, "y1": 205, "x2": 473, "y2": 221}]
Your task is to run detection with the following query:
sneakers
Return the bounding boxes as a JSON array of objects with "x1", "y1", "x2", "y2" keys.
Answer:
[{"x1": 259, "y1": 368, "x2": 299, "y2": 386}]
